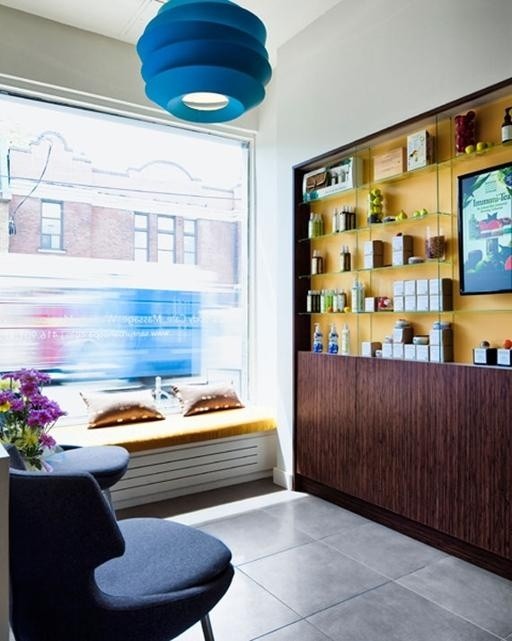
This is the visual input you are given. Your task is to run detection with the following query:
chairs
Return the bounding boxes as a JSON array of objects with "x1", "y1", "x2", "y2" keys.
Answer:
[
  {"x1": 44, "y1": 440, "x2": 130, "y2": 521},
  {"x1": 8, "y1": 475, "x2": 235, "y2": 641}
]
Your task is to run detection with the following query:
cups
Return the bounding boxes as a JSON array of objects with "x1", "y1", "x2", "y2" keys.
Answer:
[{"x1": 425, "y1": 225, "x2": 445, "y2": 263}]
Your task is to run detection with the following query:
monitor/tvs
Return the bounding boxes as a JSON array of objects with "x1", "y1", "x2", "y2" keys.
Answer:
[{"x1": 457, "y1": 162, "x2": 512, "y2": 295}]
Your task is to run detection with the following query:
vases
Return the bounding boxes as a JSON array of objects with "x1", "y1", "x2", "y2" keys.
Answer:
[{"x1": 1, "y1": 431, "x2": 27, "y2": 472}]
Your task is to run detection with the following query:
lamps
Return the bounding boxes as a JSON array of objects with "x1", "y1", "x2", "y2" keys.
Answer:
[{"x1": 136, "y1": 0, "x2": 272, "y2": 124}]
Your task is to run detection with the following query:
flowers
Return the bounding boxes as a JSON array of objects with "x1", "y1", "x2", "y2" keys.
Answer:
[{"x1": 0, "y1": 367, "x2": 67, "y2": 448}]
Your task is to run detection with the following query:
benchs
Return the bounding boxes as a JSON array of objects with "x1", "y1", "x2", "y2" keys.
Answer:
[{"x1": 58, "y1": 409, "x2": 278, "y2": 512}]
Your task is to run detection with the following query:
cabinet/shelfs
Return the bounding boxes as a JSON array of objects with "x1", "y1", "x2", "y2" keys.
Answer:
[{"x1": 294, "y1": 75, "x2": 512, "y2": 582}]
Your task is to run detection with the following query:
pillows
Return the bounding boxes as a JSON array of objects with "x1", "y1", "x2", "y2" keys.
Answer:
[{"x1": 79, "y1": 380, "x2": 245, "y2": 429}]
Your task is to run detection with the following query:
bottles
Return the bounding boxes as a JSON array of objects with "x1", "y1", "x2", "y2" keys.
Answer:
[
  {"x1": 339, "y1": 244, "x2": 351, "y2": 271},
  {"x1": 312, "y1": 249, "x2": 323, "y2": 275},
  {"x1": 307, "y1": 288, "x2": 347, "y2": 313},
  {"x1": 351, "y1": 281, "x2": 365, "y2": 313},
  {"x1": 332, "y1": 205, "x2": 355, "y2": 234},
  {"x1": 308, "y1": 211, "x2": 323, "y2": 240}
]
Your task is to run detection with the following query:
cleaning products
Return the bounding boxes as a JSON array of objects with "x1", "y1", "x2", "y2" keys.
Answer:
[
  {"x1": 313, "y1": 322, "x2": 323, "y2": 353},
  {"x1": 328, "y1": 324, "x2": 338, "y2": 354},
  {"x1": 306, "y1": 207, "x2": 365, "y2": 314}
]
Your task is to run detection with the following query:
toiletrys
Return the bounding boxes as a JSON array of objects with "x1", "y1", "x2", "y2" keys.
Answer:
[
  {"x1": 306, "y1": 205, "x2": 366, "y2": 314},
  {"x1": 341, "y1": 324, "x2": 353, "y2": 356},
  {"x1": 501, "y1": 107, "x2": 512, "y2": 143}
]
